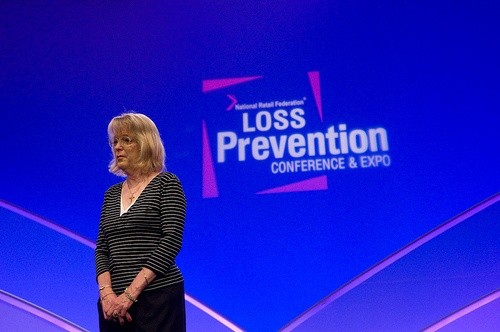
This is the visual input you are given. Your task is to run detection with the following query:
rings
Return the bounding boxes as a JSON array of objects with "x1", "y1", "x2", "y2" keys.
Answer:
[{"x1": 114, "y1": 312, "x2": 119, "y2": 317}]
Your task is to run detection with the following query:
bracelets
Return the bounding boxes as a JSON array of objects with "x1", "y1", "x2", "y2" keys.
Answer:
[
  {"x1": 100, "y1": 292, "x2": 115, "y2": 301},
  {"x1": 141, "y1": 267, "x2": 149, "y2": 285},
  {"x1": 124, "y1": 289, "x2": 137, "y2": 302},
  {"x1": 99, "y1": 284, "x2": 111, "y2": 290}
]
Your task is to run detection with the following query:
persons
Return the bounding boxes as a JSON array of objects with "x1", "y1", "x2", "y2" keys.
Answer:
[{"x1": 95, "y1": 113, "x2": 188, "y2": 332}]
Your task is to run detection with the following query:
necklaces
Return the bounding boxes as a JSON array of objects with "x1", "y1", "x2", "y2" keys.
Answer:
[{"x1": 126, "y1": 174, "x2": 149, "y2": 202}]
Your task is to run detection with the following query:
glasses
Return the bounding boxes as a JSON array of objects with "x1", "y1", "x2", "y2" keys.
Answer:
[{"x1": 108, "y1": 136, "x2": 135, "y2": 147}]
